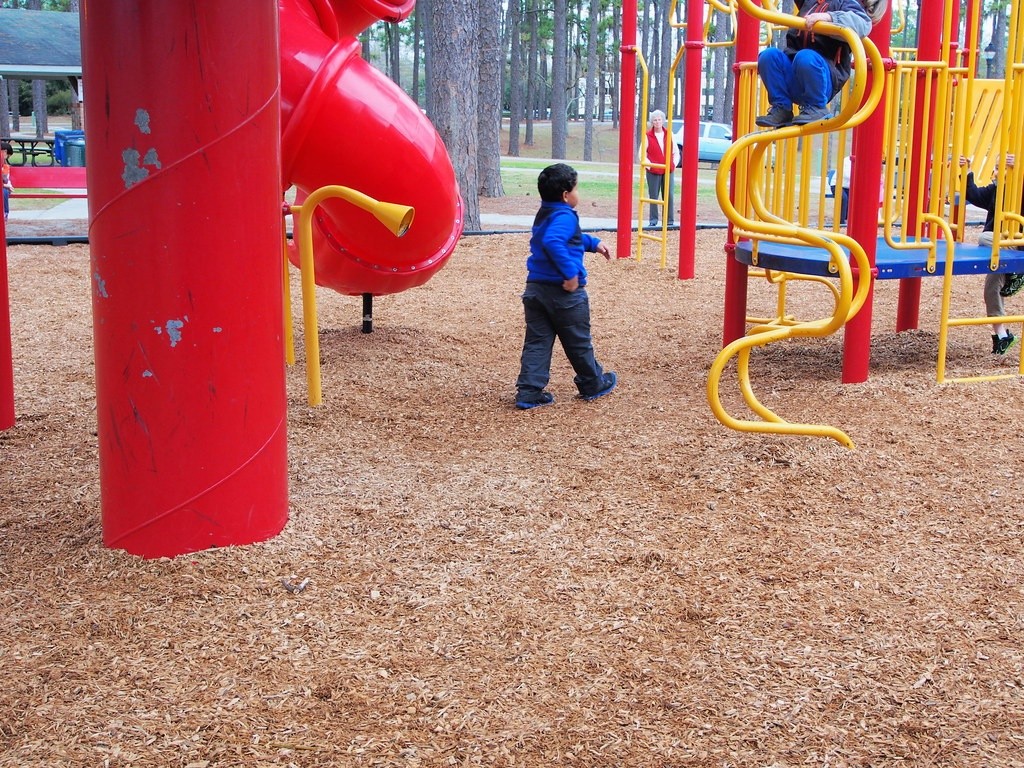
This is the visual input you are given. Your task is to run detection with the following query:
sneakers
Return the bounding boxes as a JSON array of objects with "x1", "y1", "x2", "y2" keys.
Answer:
[
  {"x1": 756, "y1": 107, "x2": 793, "y2": 126},
  {"x1": 792, "y1": 103, "x2": 833, "y2": 122},
  {"x1": 580, "y1": 372, "x2": 617, "y2": 400},
  {"x1": 991, "y1": 329, "x2": 1018, "y2": 355},
  {"x1": 1000, "y1": 273, "x2": 1024, "y2": 296},
  {"x1": 516, "y1": 390, "x2": 552, "y2": 408}
]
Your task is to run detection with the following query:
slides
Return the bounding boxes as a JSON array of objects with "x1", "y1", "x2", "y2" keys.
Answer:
[{"x1": 276, "y1": 0, "x2": 465, "y2": 297}]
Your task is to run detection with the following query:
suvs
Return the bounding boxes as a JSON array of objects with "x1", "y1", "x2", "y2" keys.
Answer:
[{"x1": 651, "y1": 120, "x2": 781, "y2": 169}]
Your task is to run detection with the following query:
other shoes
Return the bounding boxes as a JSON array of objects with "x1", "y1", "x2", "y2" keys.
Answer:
[{"x1": 650, "y1": 224, "x2": 655, "y2": 226}]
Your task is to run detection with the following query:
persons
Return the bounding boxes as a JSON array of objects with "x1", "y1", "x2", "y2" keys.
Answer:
[
  {"x1": 637, "y1": 109, "x2": 681, "y2": 229},
  {"x1": 0, "y1": 141, "x2": 14, "y2": 219},
  {"x1": 830, "y1": 150, "x2": 854, "y2": 225},
  {"x1": 514, "y1": 163, "x2": 616, "y2": 410},
  {"x1": 754, "y1": 0, "x2": 891, "y2": 128},
  {"x1": 958, "y1": 146, "x2": 1024, "y2": 356}
]
